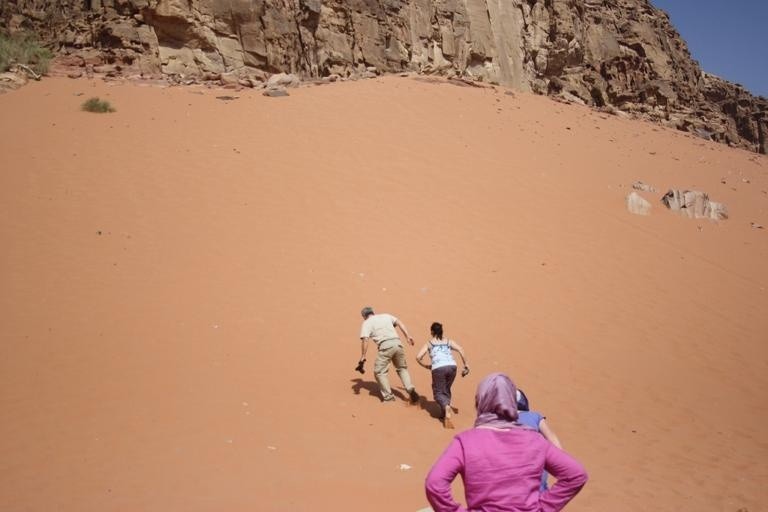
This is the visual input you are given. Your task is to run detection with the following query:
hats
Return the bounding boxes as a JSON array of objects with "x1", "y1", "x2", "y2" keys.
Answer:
[{"x1": 361, "y1": 306, "x2": 373, "y2": 316}]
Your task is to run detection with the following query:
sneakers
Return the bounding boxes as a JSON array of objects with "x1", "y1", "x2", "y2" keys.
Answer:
[
  {"x1": 444, "y1": 404, "x2": 452, "y2": 418},
  {"x1": 410, "y1": 390, "x2": 418, "y2": 401},
  {"x1": 383, "y1": 396, "x2": 395, "y2": 401}
]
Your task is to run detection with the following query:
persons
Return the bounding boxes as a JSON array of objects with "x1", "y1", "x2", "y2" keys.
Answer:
[
  {"x1": 359, "y1": 307, "x2": 419, "y2": 403},
  {"x1": 416, "y1": 322, "x2": 470, "y2": 429},
  {"x1": 515, "y1": 388, "x2": 562, "y2": 495},
  {"x1": 424, "y1": 371, "x2": 589, "y2": 512}
]
